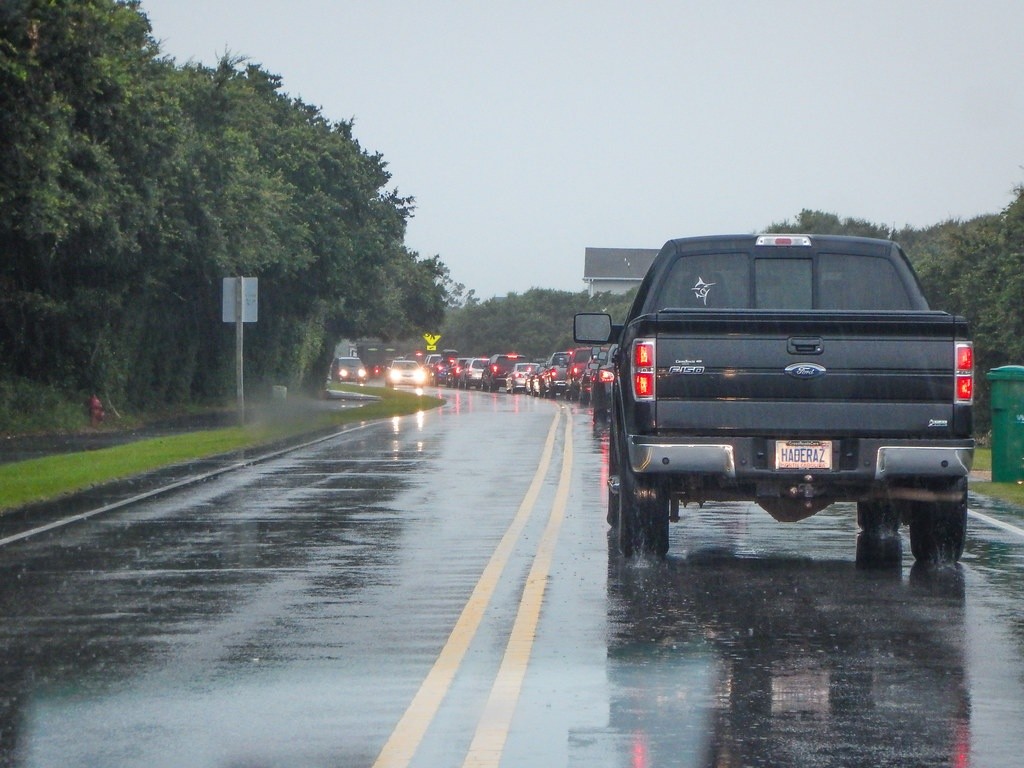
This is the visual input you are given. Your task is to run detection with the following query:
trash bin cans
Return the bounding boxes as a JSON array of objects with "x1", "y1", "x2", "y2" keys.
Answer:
[{"x1": 986, "y1": 365, "x2": 1024, "y2": 483}]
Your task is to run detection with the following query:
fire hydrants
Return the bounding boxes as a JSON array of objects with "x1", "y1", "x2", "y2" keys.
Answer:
[{"x1": 90, "y1": 397, "x2": 106, "y2": 426}]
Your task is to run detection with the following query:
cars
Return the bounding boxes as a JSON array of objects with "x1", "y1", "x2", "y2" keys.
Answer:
[
  {"x1": 384, "y1": 360, "x2": 425, "y2": 388},
  {"x1": 403, "y1": 352, "x2": 424, "y2": 364},
  {"x1": 582, "y1": 347, "x2": 609, "y2": 402},
  {"x1": 331, "y1": 357, "x2": 368, "y2": 384},
  {"x1": 538, "y1": 352, "x2": 573, "y2": 399},
  {"x1": 445, "y1": 358, "x2": 474, "y2": 388},
  {"x1": 565, "y1": 348, "x2": 591, "y2": 400},
  {"x1": 425, "y1": 354, "x2": 443, "y2": 364},
  {"x1": 592, "y1": 344, "x2": 618, "y2": 422},
  {"x1": 525, "y1": 366, "x2": 547, "y2": 397},
  {"x1": 423, "y1": 350, "x2": 458, "y2": 387},
  {"x1": 504, "y1": 363, "x2": 541, "y2": 394},
  {"x1": 482, "y1": 354, "x2": 527, "y2": 392},
  {"x1": 456, "y1": 358, "x2": 490, "y2": 390}
]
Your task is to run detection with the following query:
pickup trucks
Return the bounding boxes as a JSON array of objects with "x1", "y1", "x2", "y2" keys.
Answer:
[{"x1": 573, "y1": 233, "x2": 976, "y2": 564}]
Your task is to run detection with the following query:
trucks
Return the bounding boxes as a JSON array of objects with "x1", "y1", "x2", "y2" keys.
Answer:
[{"x1": 357, "y1": 341, "x2": 396, "y2": 377}]
